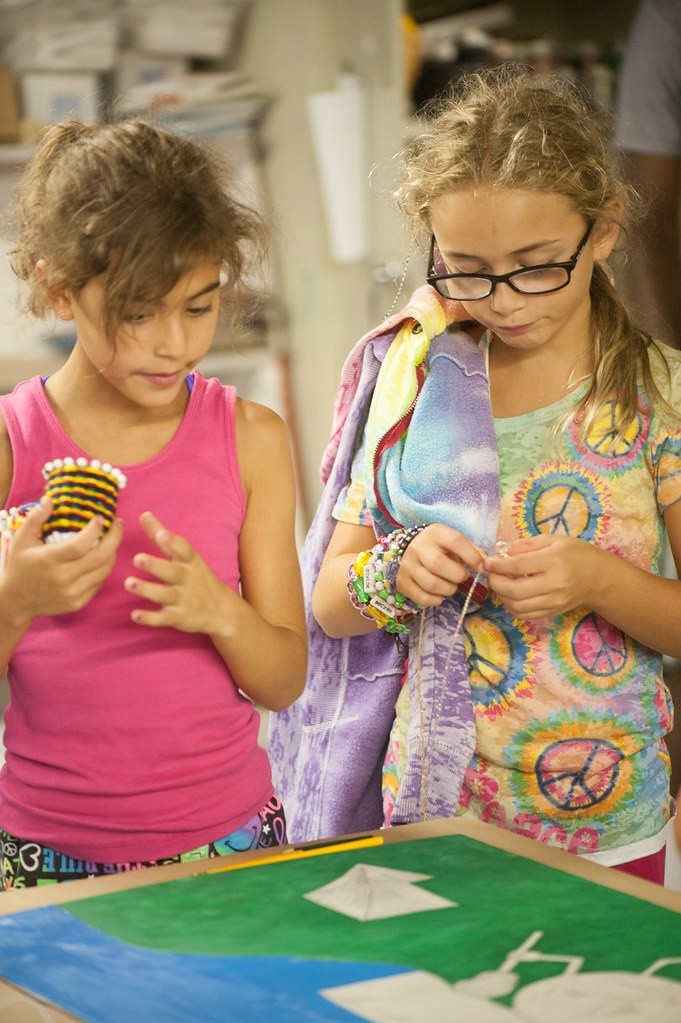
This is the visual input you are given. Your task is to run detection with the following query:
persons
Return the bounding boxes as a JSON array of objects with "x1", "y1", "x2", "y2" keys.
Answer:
[
  {"x1": 0, "y1": 118, "x2": 306, "y2": 890},
  {"x1": 269, "y1": 81, "x2": 681, "y2": 887}
]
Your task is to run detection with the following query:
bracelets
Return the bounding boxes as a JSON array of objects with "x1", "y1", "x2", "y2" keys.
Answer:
[{"x1": 346, "y1": 524, "x2": 427, "y2": 635}]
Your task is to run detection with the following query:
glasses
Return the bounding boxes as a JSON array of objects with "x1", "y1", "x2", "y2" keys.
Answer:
[{"x1": 425, "y1": 219, "x2": 594, "y2": 301}]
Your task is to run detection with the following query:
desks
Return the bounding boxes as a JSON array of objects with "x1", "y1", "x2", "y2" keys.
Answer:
[{"x1": 0, "y1": 812, "x2": 681, "y2": 1023}]
